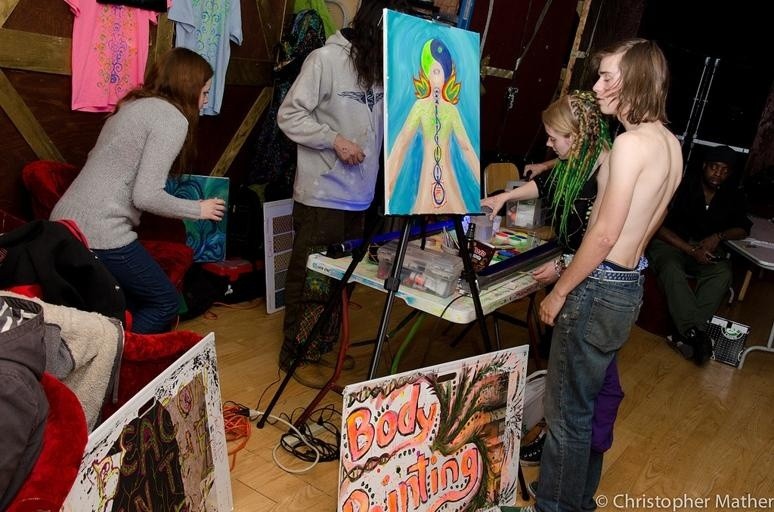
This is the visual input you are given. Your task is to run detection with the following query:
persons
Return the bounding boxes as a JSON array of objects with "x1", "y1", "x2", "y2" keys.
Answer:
[
  {"x1": 471, "y1": 90, "x2": 624, "y2": 466},
  {"x1": 276, "y1": 0, "x2": 438, "y2": 388},
  {"x1": 652, "y1": 146, "x2": 752, "y2": 365},
  {"x1": 49, "y1": 48, "x2": 227, "y2": 335},
  {"x1": 500, "y1": 35, "x2": 679, "y2": 512}
]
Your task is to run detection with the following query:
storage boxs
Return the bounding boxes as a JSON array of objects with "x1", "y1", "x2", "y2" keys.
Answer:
[{"x1": 378, "y1": 239, "x2": 464, "y2": 297}]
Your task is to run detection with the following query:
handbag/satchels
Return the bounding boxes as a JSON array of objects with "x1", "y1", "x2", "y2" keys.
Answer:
[{"x1": 522, "y1": 370, "x2": 547, "y2": 437}]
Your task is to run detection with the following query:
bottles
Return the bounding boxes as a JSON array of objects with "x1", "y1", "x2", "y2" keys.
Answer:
[{"x1": 465, "y1": 223, "x2": 475, "y2": 259}]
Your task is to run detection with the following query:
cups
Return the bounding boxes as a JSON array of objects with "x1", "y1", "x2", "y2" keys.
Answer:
[
  {"x1": 475, "y1": 208, "x2": 492, "y2": 241},
  {"x1": 527, "y1": 231, "x2": 540, "y2": 248}
]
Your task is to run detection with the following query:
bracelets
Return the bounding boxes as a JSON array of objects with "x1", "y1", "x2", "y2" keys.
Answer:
[{"x1": 554, "y1": 255, "x2": 565, "y2": 277}]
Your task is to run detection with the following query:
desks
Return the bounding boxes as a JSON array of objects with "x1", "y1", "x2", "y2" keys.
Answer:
[
  {"x1": 726, "y1": 215, "x2": 774, "y2": 369},
  {"x1": 257, "y1": 227, "x2": 573, "y2": 433}
]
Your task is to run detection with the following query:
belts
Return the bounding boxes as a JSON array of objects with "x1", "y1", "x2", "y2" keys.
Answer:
[{"x1": 589, "y1": 269, "x2": 640, "y2": 283}]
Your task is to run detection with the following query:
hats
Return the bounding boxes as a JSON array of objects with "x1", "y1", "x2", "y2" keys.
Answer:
[{"x1": 706, "y1": 145, "x2": 737, "y2": 169}]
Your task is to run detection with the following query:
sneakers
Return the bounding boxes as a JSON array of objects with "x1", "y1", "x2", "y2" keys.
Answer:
[
  {"x1": 520, "y1": 433, "x2": 545, "y2": 466},
  {"x1": 319, "y1": 351, "x2": 354, "y2": 370},
  {"x1": 280, "y1": 356, "x2": 326, "y2": 389},
  {"x1": 667, "y1": 330, "x2": 713, "y2": 366},
  {"x1": 498, "y1": 480, "x2": 538, "y2": 512}
]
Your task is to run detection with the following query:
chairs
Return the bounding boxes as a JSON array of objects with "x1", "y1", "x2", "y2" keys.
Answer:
[
  {"x1": 0, "y1": 161, "x2": 201, "y2": 512},
  {"x1": 484, "y1": 162, "x2": 520, "y2": 225}
]
